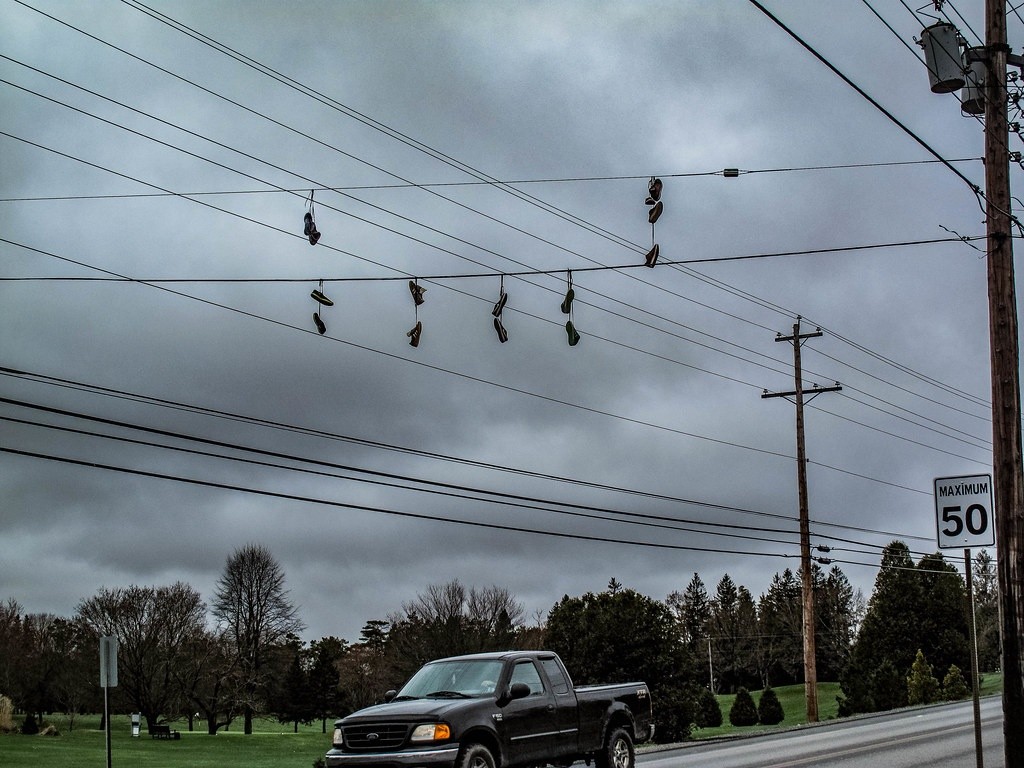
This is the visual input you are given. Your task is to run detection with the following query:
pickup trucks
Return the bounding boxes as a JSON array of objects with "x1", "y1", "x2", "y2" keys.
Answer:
[{"x1": 323, "y1": 649, "x2": 656, "y2": 768}]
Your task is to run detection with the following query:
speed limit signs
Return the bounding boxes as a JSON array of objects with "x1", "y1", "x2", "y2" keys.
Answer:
[{"x1": 933, "y1": 474, "x2": 997, "y2": 549}]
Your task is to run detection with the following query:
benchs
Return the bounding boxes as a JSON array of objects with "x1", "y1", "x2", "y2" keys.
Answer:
[{"x1": 151, "y1": 725, "x2": 175, "y2": 739}]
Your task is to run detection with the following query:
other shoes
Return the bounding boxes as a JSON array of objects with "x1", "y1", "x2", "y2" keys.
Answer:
[
  {"x1": 309, "y1": 225, "x2": 322, "y2": 245},
  {"x1": 560, "y1": 288, "x2": 574, "y2": 314},
  {"x1": 313, "y1": 312, "x2": 326, "y2": 334},
  {"x1": 493, "y1": 317, "x2": 508, "y2": 343},
  {"x1": 649, "y1": 179, "x2": 663, "y2": 201},
  {"x1": 409, "y1": 281, "x2": 427, "y2": 305},
  {"x1": 645, "y1": 197, "x2": 655, "y2": 205},
  {"x1": 311, "y1": 289, "x2": 334, "y2": 306},
  {"x1": 566, "y1": 321, "x2": 580, "y2": 346},
  {"x1": 491, "y1": 292, "x2": 508, "y2": 317},
  {"x1": 644, "y1": 244, "x2": 659, "y2": 268},
  {"x1": 648, "y1": 201, "x2": 663, "y2": 224},
  {"x1": 304, "y1": 212, "x2": 314, "y2": 235},
  {"x1": 407, "y1": 321, "x2": 422, "y2": 347}
]
